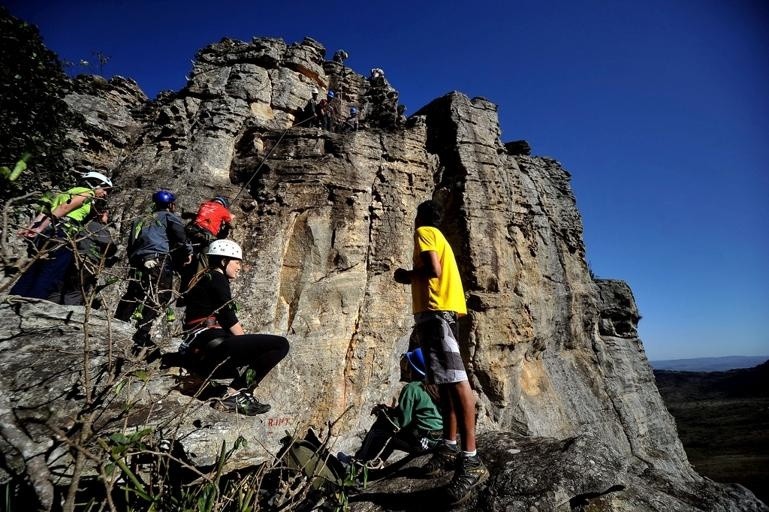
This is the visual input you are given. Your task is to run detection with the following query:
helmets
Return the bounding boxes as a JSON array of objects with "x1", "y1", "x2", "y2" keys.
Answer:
[
  {"x1": 404, "y1": 348, "x2": 428, "y2": 376},
  {"x1": 81, "y1": 171, "x2": 113, "y2": 189},
  {"x1": 205, "y1": 239, "x2": 243, "y2": 259},
  {"x1": 152, "y1": 191, "x2": 177, "y2": 204},
  {"x1": 212, "y1": 197, "x2": 227, "y2": 207},
  {"x1": 94, "y1": 199, "x2": 111, "y2": 211}
]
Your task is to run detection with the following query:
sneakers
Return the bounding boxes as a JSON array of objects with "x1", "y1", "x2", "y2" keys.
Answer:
[
  {"x1": 337, "y1": 450, "x2": 363, "y2": 474},
  {"x1": 421, "y1": 442, "x2": 460, "y2": 478},
  {"x1": 366, "y1": 457, "x2": 384, "y2": 473},
  {"x1": 195, "y1": 379, "x2": 232, "y2": 400},
  {"x1": 214, "y1": 384, "x2": 271, "y2": 416},
  {"x1": 130, "y1": 330, "x2": 157, "y2": 347},
  {"x1": 442, "y1": 454, "x2": 491, "y2": 505}
]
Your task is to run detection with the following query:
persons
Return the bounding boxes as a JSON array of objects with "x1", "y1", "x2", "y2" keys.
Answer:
[
  {"x1": 185, "y1": 238, "x2": 289, "y2": 413},
  {"x1": 47, "y1": 196, "x2": 114, "y2": 307},
  {"x1": 395, "y1": 200, "x2": 490, "y2": 499},
  {"x1": 9, "y1": 168, "x2": 114, "y2": 298},
  {"x1": 183, "y1": 196, "x2": 237, "y2": 242},
  {"x1": 129, "y1": 190, "x2": 195, "y2": 352},
  {"x1": 336, "y1": 346, "x2": 443, "y2": 475}
]
